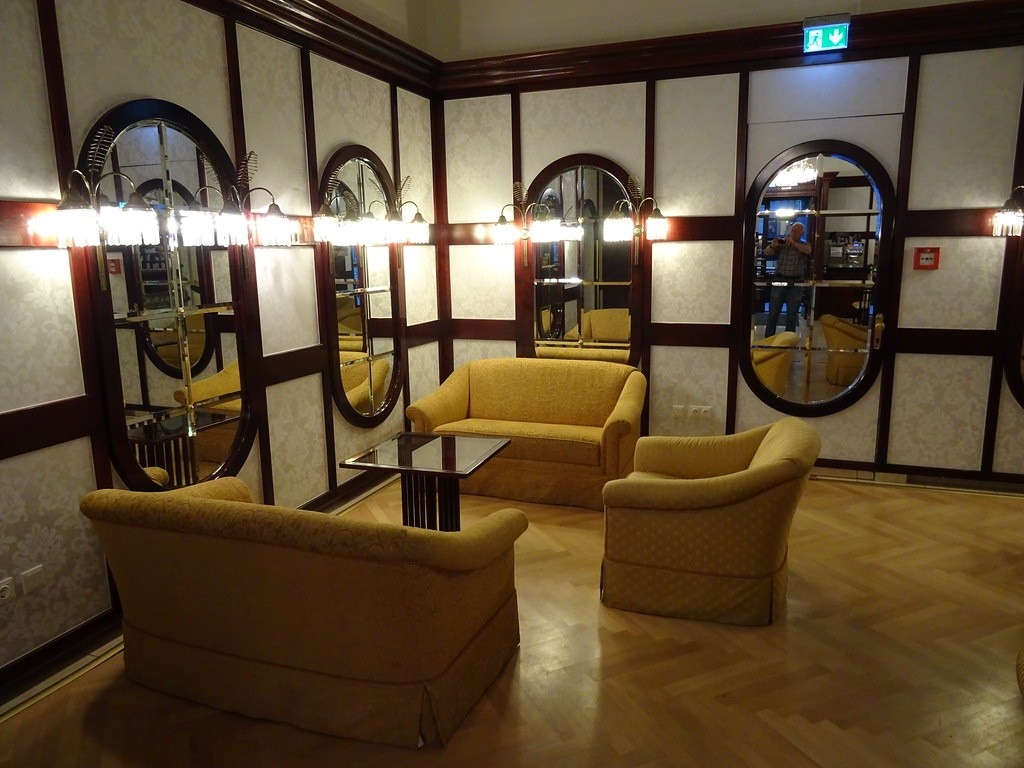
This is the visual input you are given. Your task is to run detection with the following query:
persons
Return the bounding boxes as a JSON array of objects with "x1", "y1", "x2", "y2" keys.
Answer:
[{"x1": 764, "y1": 223, "x2": 811, "y2": 337}]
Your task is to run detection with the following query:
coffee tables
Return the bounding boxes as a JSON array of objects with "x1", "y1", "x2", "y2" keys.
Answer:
[
  {"x1": 337, "y1": 431, "x2": 514, "y2": 531},
  {"x1": 122, "y1": 410, "x2": 242, "y2": 485}
]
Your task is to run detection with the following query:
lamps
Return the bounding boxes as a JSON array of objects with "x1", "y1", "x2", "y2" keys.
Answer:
[
  {"x1": 179, "y1": 185, "x2": 223, "y2": 248},
  {"x1": 986, "y1": 185, "x2": 1023, "y2": 236},
  {"x1": 51, "y1": 168, "x2": 667, "y2": 247},
  {"x1": 362, "y1": 199, "x2": 384, "y2": 244}
]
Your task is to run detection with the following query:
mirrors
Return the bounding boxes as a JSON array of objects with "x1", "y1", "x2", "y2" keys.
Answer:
[
  {"x1": 577, "y1": 198, "x2": 597, "y2": 336},
  {"x1": 524, "y1": 152, "x2": 642, "y2": 367},
  {"x1": 738, "y1": 138, "x2": 897, "y2": 418},
  {"x1": 126, "y1": 177, "x2": 213, "y2": 381},
  {"x1": 76, "y1": 98, "x2": 260, "y2": 492},
  {"x1": 318, "y1": 145, "x2": 406, "y2": 429},
  {"x1": 533, "y1": 187, "x2": 563, "y2": 339},
  {"x1": 333, "y1": 243, "x2": 367, "y2": 353}
]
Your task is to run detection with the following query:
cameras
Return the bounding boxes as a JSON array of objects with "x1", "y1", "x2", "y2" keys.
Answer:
[{"x1": 778, "y1": 239, "x2": 786, "y2": 244}]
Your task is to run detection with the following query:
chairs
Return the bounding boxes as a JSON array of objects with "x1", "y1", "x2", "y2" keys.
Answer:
[{"x1": 851, "y1": 288, "x2": 870, "y2": 326}]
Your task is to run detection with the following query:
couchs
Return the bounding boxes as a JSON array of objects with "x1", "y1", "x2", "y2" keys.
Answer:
[
  {"x1": 171, "y1": 359, "x2": 245, "y2": 464},
  {"x1": 596, "y1": 416, "x2": 819, "y2": 624},
  {"x1": 405, "y1": 355, "x2": 648, "y2": 512},
  {"x1": 79, "y1": 476, "x2": 533, "y2": 750},
  {"x1": 565, "y1": 306, "x2": 632, "y2": 341},
  {"x1": 337, "y1": 352, "x2": 391, "y2": 415},
  {"x1": 819, "y1": 315, "x2": 869, "y2": 385},
  {"x1": 752, "y1": 330, "x2": 800, "y2": 396}
]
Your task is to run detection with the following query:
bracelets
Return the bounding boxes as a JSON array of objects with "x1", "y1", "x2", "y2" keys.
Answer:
[{"x1": 770, "y1": 244, "x2": 775, "y2": 249}]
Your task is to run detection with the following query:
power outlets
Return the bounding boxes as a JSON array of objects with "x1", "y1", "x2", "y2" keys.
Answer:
[
  {"x1": 0, "y1": 576, "x2": 16, "y2": 606},
  {"x1": 688, "y1": 406, "x2": 712, "y2": 419}
]
